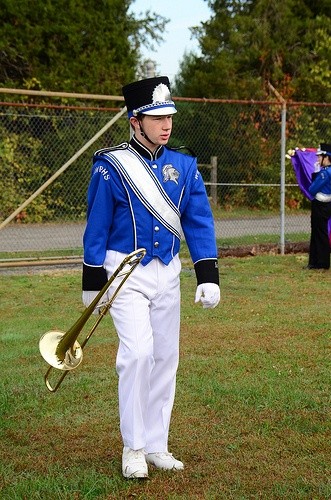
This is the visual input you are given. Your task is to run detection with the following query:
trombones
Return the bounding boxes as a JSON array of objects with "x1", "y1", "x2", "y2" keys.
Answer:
[{"x1": 38, "y1": 248, "x2": 147, "y2": 393}]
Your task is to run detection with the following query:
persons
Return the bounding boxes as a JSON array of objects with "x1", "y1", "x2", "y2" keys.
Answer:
[
  {"x1": 82, "y1": 76, "x2": 221, "y2": 478},
  {"x1": 307, "y1": 143, "x2": 331, "y2": 270}
]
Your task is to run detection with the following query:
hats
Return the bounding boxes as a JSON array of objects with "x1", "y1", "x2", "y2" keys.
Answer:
[
  {"x1": 121, "y1": 76, "x2": 178, "y2": 119},
  {"x1": 316, "y1": 143, "x2": 331, "y2": 156}
]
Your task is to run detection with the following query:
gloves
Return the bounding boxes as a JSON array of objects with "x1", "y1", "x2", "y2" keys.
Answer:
[
  {"x1": 314, "y1": 161, "x2": 320, "y2": 173},
  {"x1": 195, "y1": 282, "x2": 222, "y2": 309},
  {"x1": 82, "y1": 291, "x2": 107, "y2": 315}
]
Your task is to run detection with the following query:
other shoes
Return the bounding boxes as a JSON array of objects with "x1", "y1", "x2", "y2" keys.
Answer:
[
  {"x1": 146, "y1": 453, "x2": 184, "y2": 471},
  {"x1": 122, "y1": 447, "x2": 148, "y2": 478}
]
